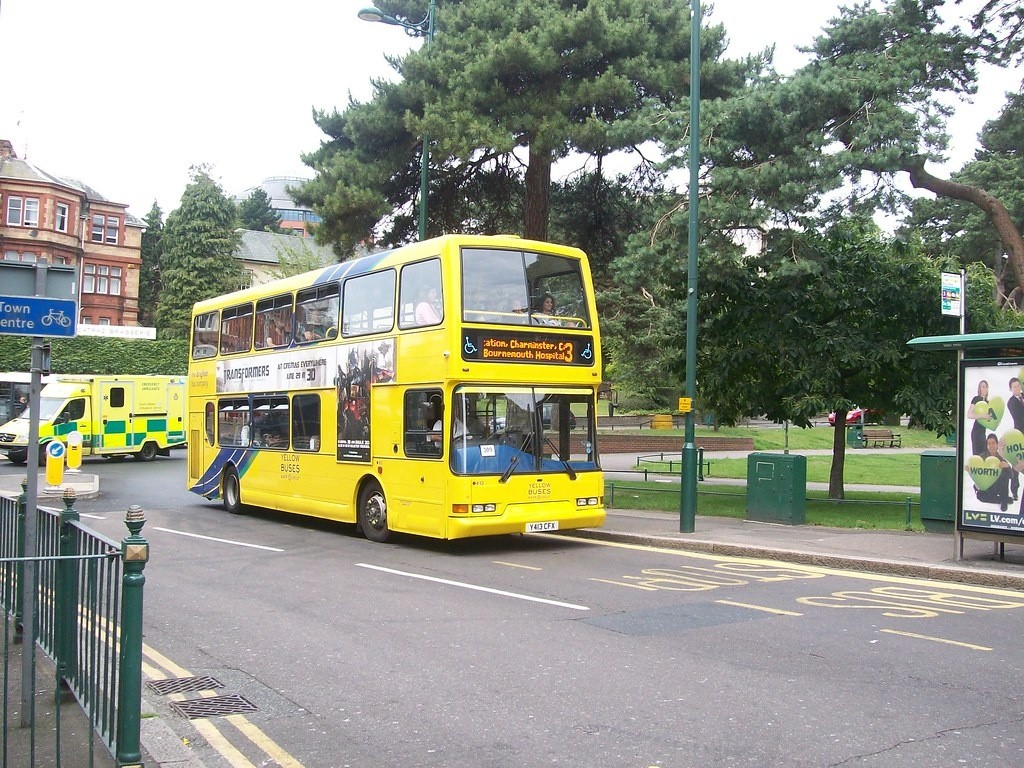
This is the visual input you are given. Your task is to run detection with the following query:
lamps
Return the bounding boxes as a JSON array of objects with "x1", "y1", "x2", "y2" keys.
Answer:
[{"x1": 141, "y1": 228, "x2": 146, "y2": 233}]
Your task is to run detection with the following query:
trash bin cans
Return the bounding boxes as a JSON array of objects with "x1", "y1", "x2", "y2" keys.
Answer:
[{"x1": 846, "y1": 423, "x2": 864, "y2": 448}]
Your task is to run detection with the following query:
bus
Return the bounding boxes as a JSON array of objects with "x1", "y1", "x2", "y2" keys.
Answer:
[{"x1": 183, "y1": 235, "x2": 618, "y2": 545}]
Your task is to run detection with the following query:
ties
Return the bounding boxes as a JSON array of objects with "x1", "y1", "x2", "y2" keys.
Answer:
[
  {"x1": 1019, "y1": 396, "x2": 1024, "y2": 407},
  {"x1": 455, "y1": 422, "x2": 458, "y2": 434}
]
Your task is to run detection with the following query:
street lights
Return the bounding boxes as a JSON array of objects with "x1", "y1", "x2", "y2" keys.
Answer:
[{"x1": 358, "y1": 0, "x2": 430, "y2": 244}]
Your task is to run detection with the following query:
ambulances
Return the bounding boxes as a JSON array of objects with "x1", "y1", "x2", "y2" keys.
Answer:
[{"x1": 0, "y1": 375, "x2": 192, "y2": 465}]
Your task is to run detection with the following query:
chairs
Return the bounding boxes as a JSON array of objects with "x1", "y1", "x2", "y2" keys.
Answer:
[{"x1": 242, "y1": 425, "x2": 319, "y2": 449}]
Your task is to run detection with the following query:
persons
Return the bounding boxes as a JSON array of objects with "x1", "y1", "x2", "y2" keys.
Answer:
[
  {"x1": 431, "y1": 415, "x2": 470, "y2": 452},
  {"x1": 998, "y1": 437, "x2": 1024, "y2": 514},
  {"x1": 503, "y1": 294, "x2": 527, "y2": 323},
  {"x1": 477, "y1": 300, "x2": 503, "y2": 322},
  {"x1": 266, "y1": 314, "x2": 286, "y2": 346},
  {"x1": 415, "y1": 285, "x2": 442, "y2": 325},
  {"x1": 302, "y1": 309, "x2": 321, "y2": 340},
  {"x1": 240, "y1": 419, "x2": 259, "y2": 446},
  {"x1": 967, "y1": 380, "x2": 997, "y2": 455},
  {"x1": 1007, "y1": 377, "x2": 1024, "y2": 434},
  {"x1": 965, "y1": 434, "x2": 1013, "y2": 511},
  {"x1": 260, "y1": 429, "x2": 284, "y2": 447},
  {"x1": 530, "y1": 294, "x2": 575, "y2": 326}
]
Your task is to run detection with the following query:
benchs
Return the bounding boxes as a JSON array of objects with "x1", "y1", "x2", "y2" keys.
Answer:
[{"x1": 862, "y1": 429, "x2": 901, "y2": 448}]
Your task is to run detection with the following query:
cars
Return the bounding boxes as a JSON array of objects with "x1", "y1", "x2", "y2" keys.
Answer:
[
  {"x1": 827, "y1": 406, "x2": 884, "y2": 426},
  {"x1": 489, "y1": 402, "x2": 577, "y2": 433}
]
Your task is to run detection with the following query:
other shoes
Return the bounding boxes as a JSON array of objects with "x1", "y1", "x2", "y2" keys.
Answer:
[
  {"x1": 1001, "y1": 498, "x2": 1007, "y2": 511},
  {"x1": 1008, "y1": 498, "x2": 1013, "y2": 504},
  {"x1": 1012, "y1": 492, "x2": 1018, "y2": 501}
]
